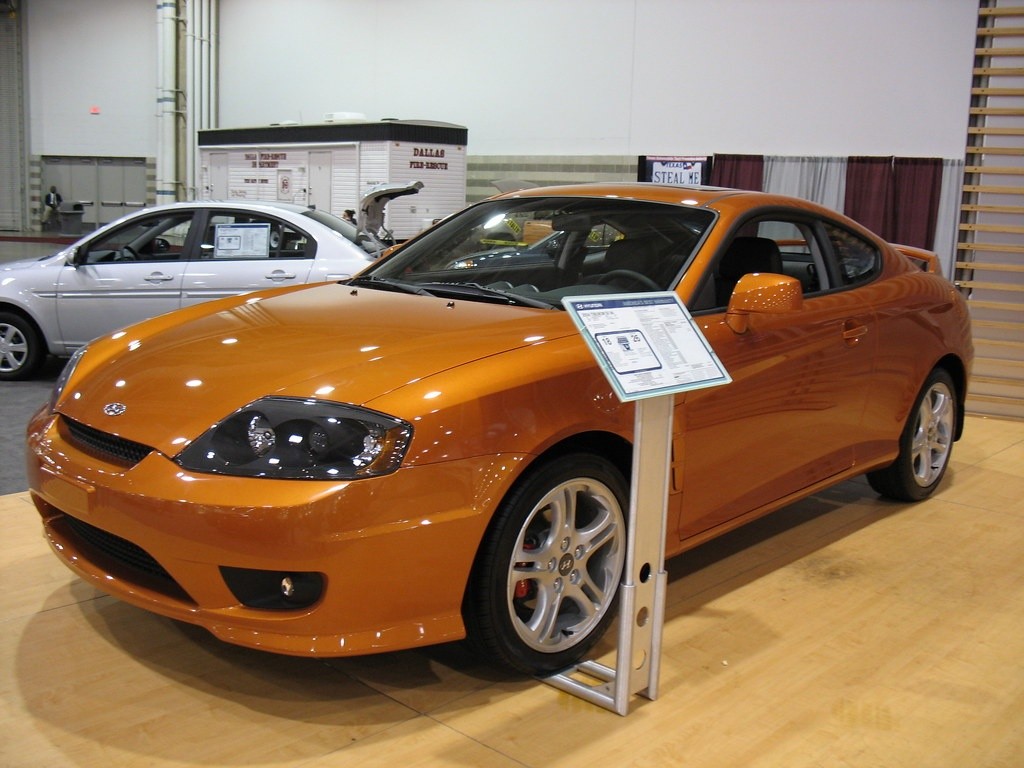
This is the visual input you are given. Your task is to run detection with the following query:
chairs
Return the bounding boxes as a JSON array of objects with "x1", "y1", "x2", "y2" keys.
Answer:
[
  {"x1": 604, "y1": 238, "x2": 659, "y2": 291},
  {"x1": 715, "y1": 235, "x2": 785, "y2": 310}
]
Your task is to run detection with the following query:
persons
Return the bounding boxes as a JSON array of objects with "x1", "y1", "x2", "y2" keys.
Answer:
[
  {"x1": 342, "y1": 209, "x2": 356, "y2": 224},
  {"x1": 41, "y1": 185, "x2": 62, "y2": 223}
]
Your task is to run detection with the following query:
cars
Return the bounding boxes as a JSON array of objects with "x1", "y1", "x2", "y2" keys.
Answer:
[
  {"x1": 1, "y1": 179, "x2": 425, "y2": 376},
  {"x1": 26, "y1": 180, "x2": 975, "y2": 675}
]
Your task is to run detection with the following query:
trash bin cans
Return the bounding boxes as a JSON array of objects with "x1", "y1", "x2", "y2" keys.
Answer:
[{"x1": 58, "y1": 201, "x2": 85, "y2": 235}]
